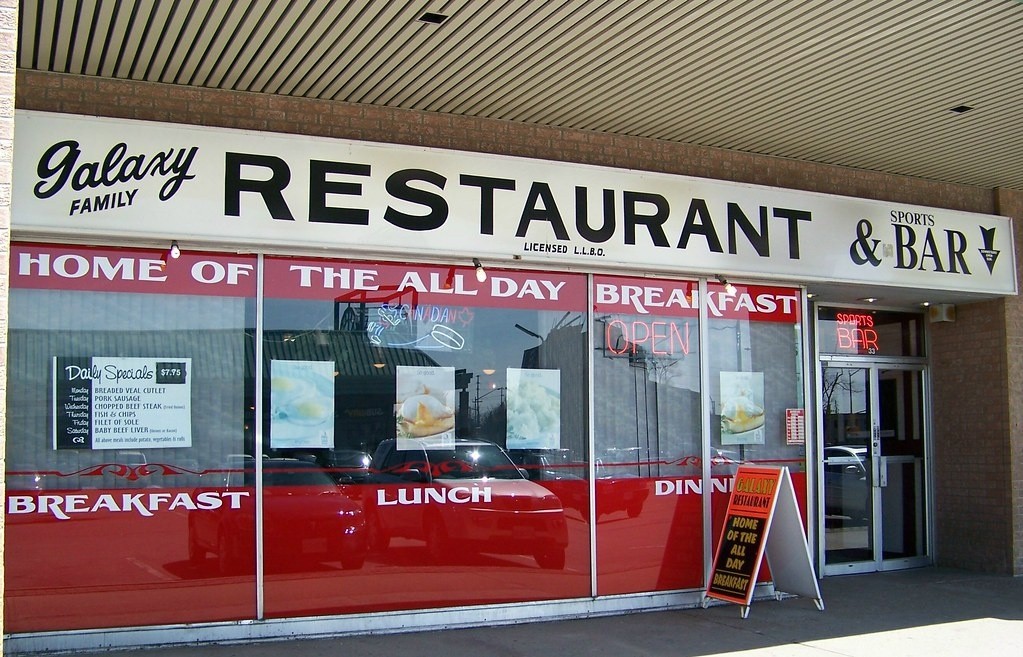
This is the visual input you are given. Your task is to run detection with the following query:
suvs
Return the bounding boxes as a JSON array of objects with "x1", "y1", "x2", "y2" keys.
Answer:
[{"x1": 110, "y1": 451, "x2": 151, "y2": 487}]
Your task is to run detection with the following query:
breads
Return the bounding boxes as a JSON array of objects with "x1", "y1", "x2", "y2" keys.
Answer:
[
  {"x1": 723, "y1": 412, "x2": 765, "y2": 433},
  {"x1": 398, "y1": 415, "x2": 455, "y2": 437}
]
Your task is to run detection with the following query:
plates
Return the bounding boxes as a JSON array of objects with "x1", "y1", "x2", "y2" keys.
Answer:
[{"x1": 396, "y1": 427, "x2": 455, "y2": 441}]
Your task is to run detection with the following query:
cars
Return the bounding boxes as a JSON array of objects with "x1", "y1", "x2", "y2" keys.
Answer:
[
  {"x1": 505, "y1": 443, "x2": 756, "y2": 523},
  {"x1": 824, "y1": 445, "x2": 869, "y2": 520},
  {"x1": 315, "y1": 450, "x2": 373, "y2": 500},
  {"x1": 43, "y1": 449, "x2": 83, "y2": 492},
  {"x1": 368, "y1": 436, "x2": 569, "y2": 570},
  {"x1": 225, "y1": 455, "x2": 253, "y2": 461},
  {"x1": 187, "y1": 460, "x2": 373, "y2": 571},
  {"x1": 6, "y1": 464, "x2": 44, "y2": 496}
]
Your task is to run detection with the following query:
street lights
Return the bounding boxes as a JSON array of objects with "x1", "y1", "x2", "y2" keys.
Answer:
[{"x1": 514, "y1": 324, "x2": 546, "y2": 369}]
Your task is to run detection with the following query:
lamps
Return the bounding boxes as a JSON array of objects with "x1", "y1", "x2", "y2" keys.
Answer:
[
  {"x1": 373, "y1": 339, "x2": 386, "y2": 369},
  {"x1": 476, "y1": 339, "x2": 498, "y2": 376},
  {"x1": 325, "y1": 354, "x2": 341, "y2": 378}
]
